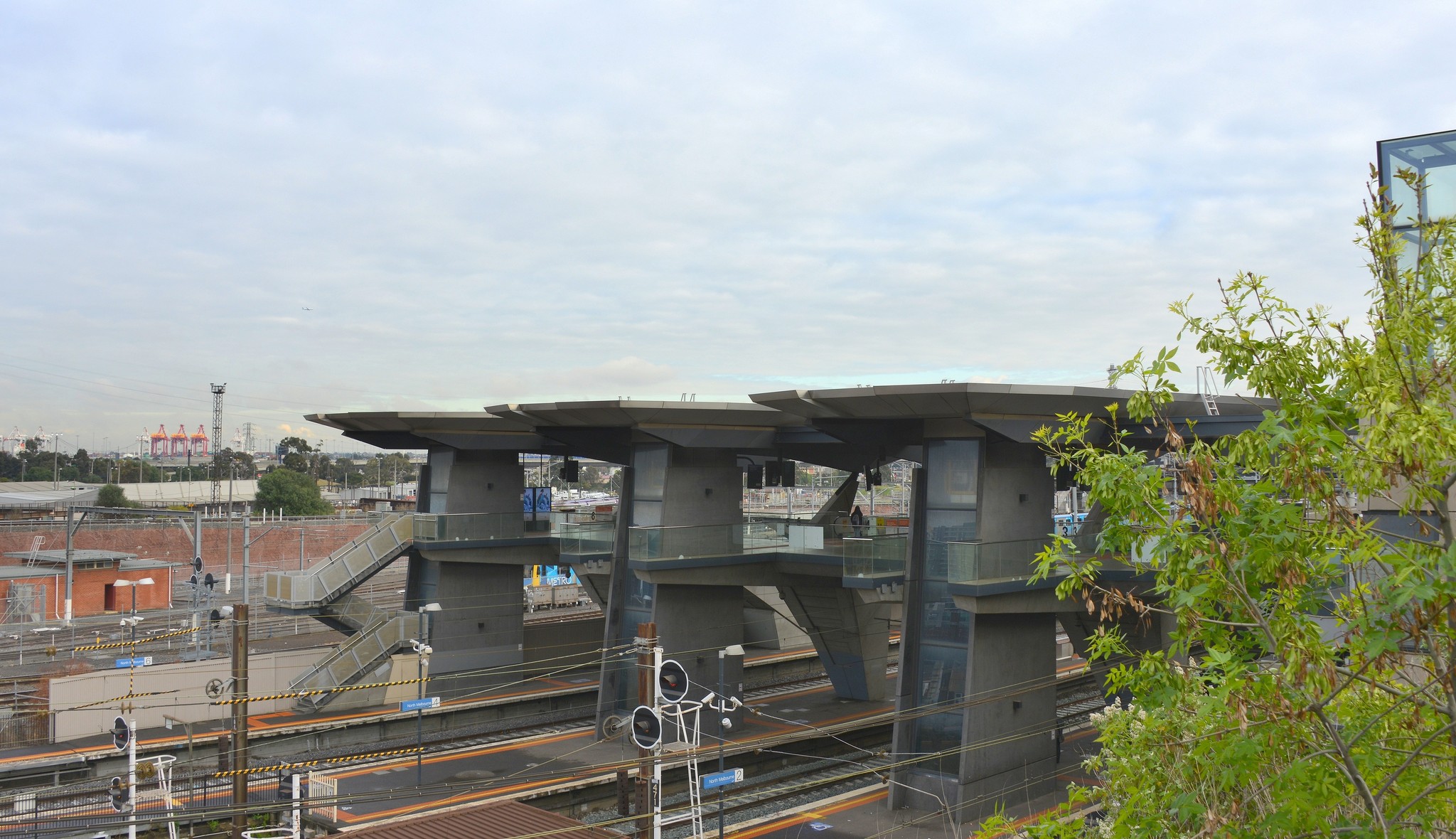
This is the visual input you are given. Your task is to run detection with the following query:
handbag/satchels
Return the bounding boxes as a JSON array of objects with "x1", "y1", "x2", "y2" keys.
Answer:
[{"x1": 1059, "y1": 734, "x2": 1066, "y2": 743}]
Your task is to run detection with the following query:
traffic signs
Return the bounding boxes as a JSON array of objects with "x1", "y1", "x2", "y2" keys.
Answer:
[
  {"x1": 699, "y1": 767, "x2": 744, "y2": 790},
  {"x1": 400, "y1": 697, "x2": 440, "y2": 712},
  {"x1": 116, "y1": 656, "x2": 152, "y2": 668}
]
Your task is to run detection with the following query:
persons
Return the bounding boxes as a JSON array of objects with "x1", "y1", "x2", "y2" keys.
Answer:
[
  {"x1": 536, "y1": 489, "x2": 549, "y2": 511},
  {"x1": 851, "y1": 506, "x2": 863, "y2": 537},
  {"x1": 524, "y1": 489, "x2": 533, "y2": 512}
]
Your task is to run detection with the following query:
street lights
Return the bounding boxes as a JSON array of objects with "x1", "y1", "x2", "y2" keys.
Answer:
[
  {"x1": 700, "y1": 644, "x2": 746, "y2": 839},
  {"x1": 410, "y1": 603, "x2": 443, "y2": 787},
  {"x1": 113, "y1": 577, "x2": 155, "y2": 659}
]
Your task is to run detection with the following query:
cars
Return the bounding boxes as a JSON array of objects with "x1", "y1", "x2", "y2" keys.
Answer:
[{"x1": 552, "y1": 487, "x2": 616, "y2": 501}]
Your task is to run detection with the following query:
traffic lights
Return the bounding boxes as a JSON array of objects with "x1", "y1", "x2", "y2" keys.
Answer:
[
  {"x1": 658, "y1": 660, "x2": 689, "y2": 703},
  {"x1": 631, "y1": 705, "x2": 662, "y2": 749},
  {"x1": 109, "y1": 716, "x2": 130, "y2": 751},
  {"x1": 190, "y1": 556, "x2": 203, "y2": 573},
  {"x1": 107, "y1": 776, "x2": 130, "y2": 813},
  {"x1": 185, "y1": 574, "x2": 199, "y2": 592}
]
